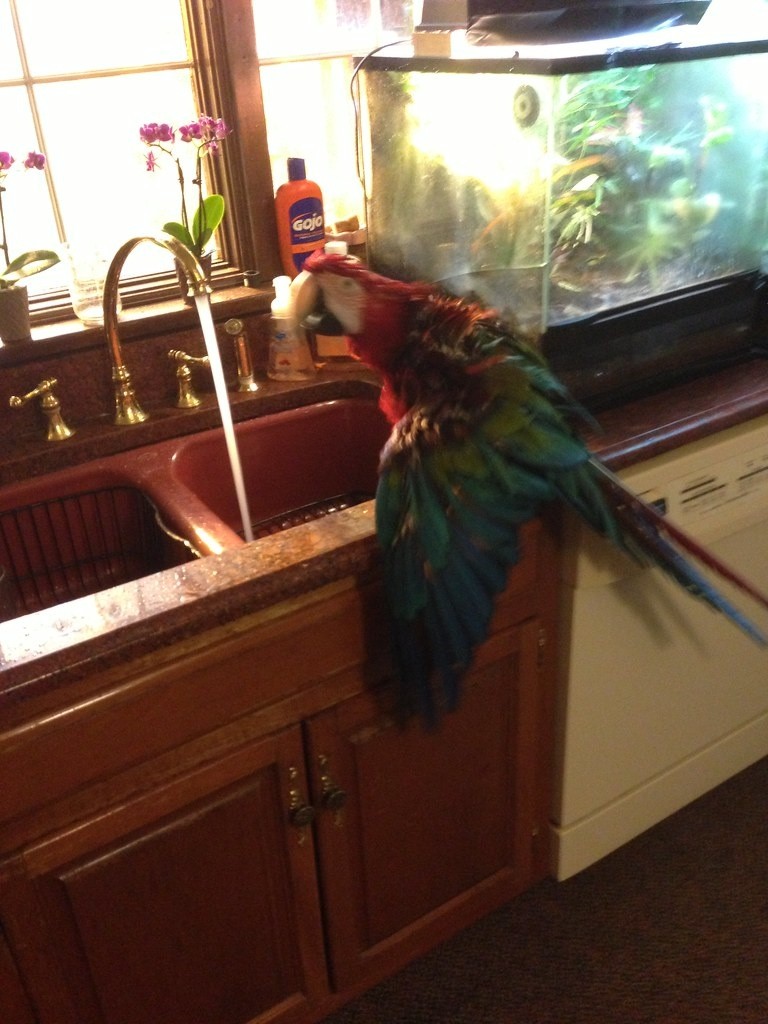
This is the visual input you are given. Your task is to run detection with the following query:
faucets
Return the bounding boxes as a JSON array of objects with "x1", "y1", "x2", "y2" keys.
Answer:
[{"x1": 103, "y1": 232, "x2": 213, "y2": 426}]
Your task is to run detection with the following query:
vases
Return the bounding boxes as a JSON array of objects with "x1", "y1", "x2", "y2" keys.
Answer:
[
  {"x1": 174, "y1": 257, "x2": 213, "y2": 307},
  {"x1": 0, "y1": 285, "x2": 31, "y2": 342}
]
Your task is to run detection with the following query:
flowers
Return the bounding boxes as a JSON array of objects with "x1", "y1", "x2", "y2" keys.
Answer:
[
  {"x1": 0, "y1": 151, "x2": 61, "y2": 288},
  {"x1": 139, "y1": 114, "x2": 231, "y2": 255}
]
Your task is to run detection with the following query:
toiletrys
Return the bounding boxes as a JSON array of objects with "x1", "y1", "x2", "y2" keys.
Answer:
[{"x1": 263, "y1": 276, "x2": 313, "y2": 380}]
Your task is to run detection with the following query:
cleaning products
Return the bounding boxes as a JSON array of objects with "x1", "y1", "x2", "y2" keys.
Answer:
[{"x1": 274, "y1": 157, "x2": 327, "y2": 274}]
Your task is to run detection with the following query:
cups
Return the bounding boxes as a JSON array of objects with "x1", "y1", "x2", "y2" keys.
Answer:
[{"x1": 56, "y1": 238, "x2": 124, "y2": 323}]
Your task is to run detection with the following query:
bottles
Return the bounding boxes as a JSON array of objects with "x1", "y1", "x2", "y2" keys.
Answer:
[
  {"x1": 321, "y1": 240, "x2": 349, "y2": 259},
  {"x1": 272, "y1": 157, "x2": 329, "y2": 282}
]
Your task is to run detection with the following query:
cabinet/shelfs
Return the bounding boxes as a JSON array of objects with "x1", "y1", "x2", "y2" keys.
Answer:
[{"x1": 0, "y1": 520, "x2": 547, "y2": 1024}]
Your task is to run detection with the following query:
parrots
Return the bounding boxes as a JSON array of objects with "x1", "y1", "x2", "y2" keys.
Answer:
[{"x1": 282, "y1": 240, "x2": 768, "y2": 738}]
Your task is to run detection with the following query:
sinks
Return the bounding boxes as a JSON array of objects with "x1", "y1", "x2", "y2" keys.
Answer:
[
  {"x1": 169, "y1": 401, "x2": 451, "y2": 547},
  {"x1": 4, "y1": 466, "x2": 212, "y2": 627}
]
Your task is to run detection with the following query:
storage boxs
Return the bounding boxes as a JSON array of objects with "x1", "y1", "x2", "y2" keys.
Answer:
[{"x1": 354, "y1": 40, "x2": 768, "y2": 418}]
